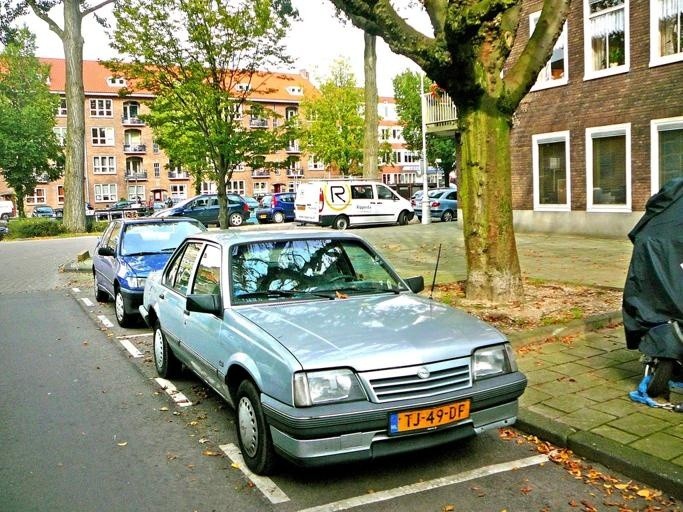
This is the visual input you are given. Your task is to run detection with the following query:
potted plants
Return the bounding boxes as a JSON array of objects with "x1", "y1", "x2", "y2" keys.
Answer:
[{"x1": 601, "y1": 46, "x2": 624, "y2": 69}]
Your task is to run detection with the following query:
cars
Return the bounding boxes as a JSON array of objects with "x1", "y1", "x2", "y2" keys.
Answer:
[
  {"x1": 32, "y1": 205, "x2": 56, "y2": 219},
  {"x1": 149, "y1": 193, "x2": 251, "y2": 228},
  {"x1": 255, "y1": 192, "x2": 297, "y2": 223},
  {"x1": 243, "y1": 197, "x2": 260, "y2": 210},
  {"x1": 409, "y1": 190, "x2": 424, "y2": 207},
  {"x1": 91, "y1": 217, "x2": 209, "y2": 328},
  {"x1": 57, "y1": 200, "x2": 156, "y2": 221},
  {"x1": 0, "y1": 201, "x2": 15, "y2": 220},
  {"x1": 139, "y1": 230, "x2": 530, "y2": 478},
  {"x1": 411, "y1": 189, "x2": 458, "y2": 223}
]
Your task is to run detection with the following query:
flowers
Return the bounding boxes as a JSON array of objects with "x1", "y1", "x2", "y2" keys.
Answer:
[{"x1": 431, "y1": 84, "x2": 445, "y2": 100}]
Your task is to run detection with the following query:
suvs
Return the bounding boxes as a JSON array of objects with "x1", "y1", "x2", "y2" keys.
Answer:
[{"x1": 293, "y1": 181, "x2": 416, "y2": 230}]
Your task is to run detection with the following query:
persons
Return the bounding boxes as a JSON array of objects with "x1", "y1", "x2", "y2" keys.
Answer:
[{"x1": 166, "y1": 197, "x2": 172, "y2": 207}]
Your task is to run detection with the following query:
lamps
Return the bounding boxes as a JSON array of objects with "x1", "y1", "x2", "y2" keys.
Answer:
[{"x1": 549, "y1": 157, "x2": 561, "y2": 192}]
